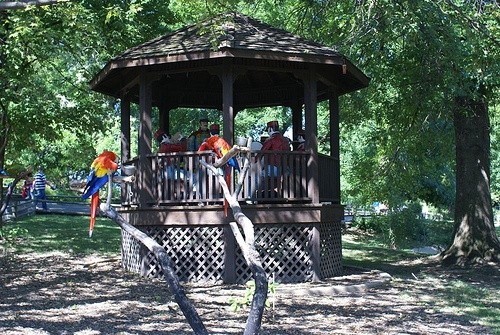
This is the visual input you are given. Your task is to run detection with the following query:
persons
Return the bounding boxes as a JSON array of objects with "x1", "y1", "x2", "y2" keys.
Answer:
[
  {"x1": 24, "y1": 177, "x2": 35, "y2": 201},
  {"x1": 245, "y1": 121, "x2": 305, "y2": 205},
  {"x1": 31, "y1": 165, "x2": 56, "y2": 210},
  {"x1": 154, "y1": 117, "x2": 240, "y2": 204}
]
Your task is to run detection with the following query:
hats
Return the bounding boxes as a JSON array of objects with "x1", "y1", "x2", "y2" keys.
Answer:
[
  {"x1": 154, "y1": 127, "x2": 166, "y2": 139},
  {"x1": 264, "y1": 121, "x2": 279, "y2": 132},
  {"x1": 208, "y1": 124, "x2": 219, "y2": 132},
  {"x1": 198, "y1": 115, "x2": 210, "y2": 122}
]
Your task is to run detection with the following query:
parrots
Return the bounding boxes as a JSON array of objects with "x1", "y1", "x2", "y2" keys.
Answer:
[
  {"x1": 80, "y1": 149, "x2": 120, "y2": 237},
  {"x1": 198, "y1": 135, "x2": 241, "y2": 219}
]
